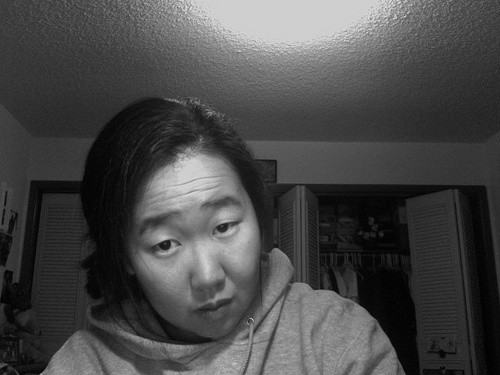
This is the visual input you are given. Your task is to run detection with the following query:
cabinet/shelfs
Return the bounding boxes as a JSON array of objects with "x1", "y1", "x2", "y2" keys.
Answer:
[{"x1": 278, "y1": 186, "x2": 491, "y2": 375}]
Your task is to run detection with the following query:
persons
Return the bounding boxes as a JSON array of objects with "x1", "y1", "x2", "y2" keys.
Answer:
[{"x1": 38, "y1": 96, "x2": 404, "y2": 375}]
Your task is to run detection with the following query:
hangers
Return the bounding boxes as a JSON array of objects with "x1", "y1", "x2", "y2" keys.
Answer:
[{"x1": 320, "y1": 253, "x2": 411, "y2": 272}]
